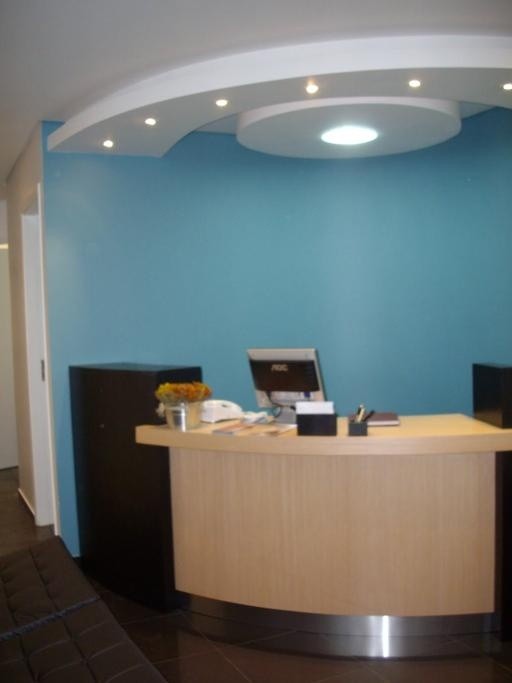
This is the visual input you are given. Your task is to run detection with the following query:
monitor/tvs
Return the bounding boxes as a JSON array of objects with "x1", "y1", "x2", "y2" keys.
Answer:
[{"x1": 245, "y1": 347, "x2": 328, "y2": 429}]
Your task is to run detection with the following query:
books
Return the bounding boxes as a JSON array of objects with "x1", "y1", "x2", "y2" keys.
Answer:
[
  {"x1": 349, "y1": 411, "x2": 401, "y2": 426},
  {"x1": 212, "y1": 421, "x2": 297, "y2": 437}
]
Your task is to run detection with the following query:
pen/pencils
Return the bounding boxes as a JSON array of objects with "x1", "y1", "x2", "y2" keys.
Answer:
[{"x1": 355, "y1": 404, "x2": 375, "y2": 423}]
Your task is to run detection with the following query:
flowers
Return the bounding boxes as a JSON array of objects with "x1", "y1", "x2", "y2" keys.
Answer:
[{"x1": 155, "y1": 379, "x2": 211, "y2": 403}]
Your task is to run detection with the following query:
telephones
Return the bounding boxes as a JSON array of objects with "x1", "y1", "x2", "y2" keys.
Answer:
[{"x1": 200, "y1": 399, "x2": 244, "y2": 422}]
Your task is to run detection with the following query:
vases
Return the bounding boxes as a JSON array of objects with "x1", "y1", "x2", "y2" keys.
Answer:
[{"x1": 165, "y1": 401, "x2": 202, "y2": 430}]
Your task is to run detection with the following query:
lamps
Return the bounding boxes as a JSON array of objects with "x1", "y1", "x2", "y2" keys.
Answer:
[{"x1": 235, "y1": 97, "x2": 463, "y2": 159}]
